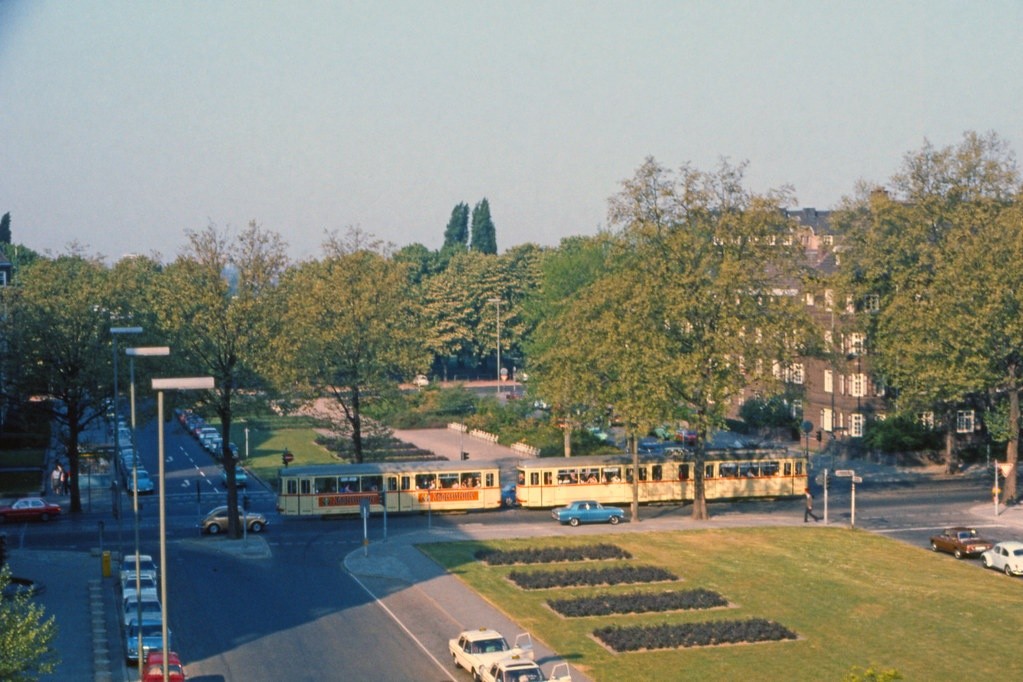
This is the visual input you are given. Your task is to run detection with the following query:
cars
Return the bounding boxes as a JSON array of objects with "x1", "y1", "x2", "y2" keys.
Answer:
[
  {"x1": 173, "y1": 406, "x2": 240, "y2": 462},
  {"x1": 979, "y1": 540, "x2": 1023, "y2": 577},
  {"x1": 413, "y1": 375, "x2": 429, "y2": 387},
  {"x1": 929, "y1": 528, "x2": 992, "y2": 559},
  {"x1": 551, "y1": 499, "x2": 628, "y2": 527},
  {"x1": 102, "y1": 398, "x2": 145, "y2": 482},
  {"x1": 222, "y1": 465, "x2": 250, "y2": 487},
  {"x1": 535, "y1": 397, "x2": 699, "y2": 444},
  {"x1": 195, "y1": 503, "x2": 272, "y2": 533},
  {"x1": 119, "y1": 553, "x2": 185, "y2": 682},
  {"x1": 0, "y1": 497, "x2": 63, "y2": 525},
  {"x1": 126, "y1": 470, "x2": 155, "y2": 495},
  {"x1": 501, "y1": 483, "x2": 517, "y2": 507},
  {"x1": 449, "y1": 626, "x2": 573, "y2": 682}
]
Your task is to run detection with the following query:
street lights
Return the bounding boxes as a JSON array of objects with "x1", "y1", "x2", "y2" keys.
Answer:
[
  {"x1": 151, "y1": 376, "x2": 216, "y2": 682},
  {"x1": 102, "y1": 326, "x2": 144, "y2": 514},
  {"x1": 125, "y1": 346, "x2": 170, "y2": 682}
]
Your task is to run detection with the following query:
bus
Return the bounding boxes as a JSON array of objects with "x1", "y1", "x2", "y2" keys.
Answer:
[
  {"x1": 516, "y1": 449, "x2": 810, "y2": 512},
  {"x1": 277, "y1": 462, "x2": 501, "y2": 517}
]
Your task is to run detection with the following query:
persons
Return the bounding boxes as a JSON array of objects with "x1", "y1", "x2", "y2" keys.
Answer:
[
  {"x1": 563, "y1": 476, "x2": 571, "y2": 484},
  {"x1": 746, "y1": 469, "x2": 755, "y2": 477},
  {"x1": 416, "y1": 479, "x2": 436, "y2": 489},
  {"x1": 602, "y1": 473, "x2": 620, "y2": 482},
  {"x1": 52, "y1": 467, "x2": 68, "y2": 496},
  {"x1": 804, "y1": 488, "x2": 818, "y2": 522},
  {"x1": 451, "y1": 479, "x2": 468, "y2": 488},
  {"x1": 476, "y1": 479, "x2": 481, "y2": 487},
  {"x1": 345, "y1": 484, "x2": 352, "y2": 492},
  {"x1": 734, "y1": 469, "x2": 741, "y2": 477},
  {"x1": 580, "y1": 473, "x2": 597, "y2": 483}
]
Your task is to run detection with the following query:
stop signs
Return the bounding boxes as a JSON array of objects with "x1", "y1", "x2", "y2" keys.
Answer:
[{"x1": 284, "y1": 454, "x2": 294, "y2": 463}]
